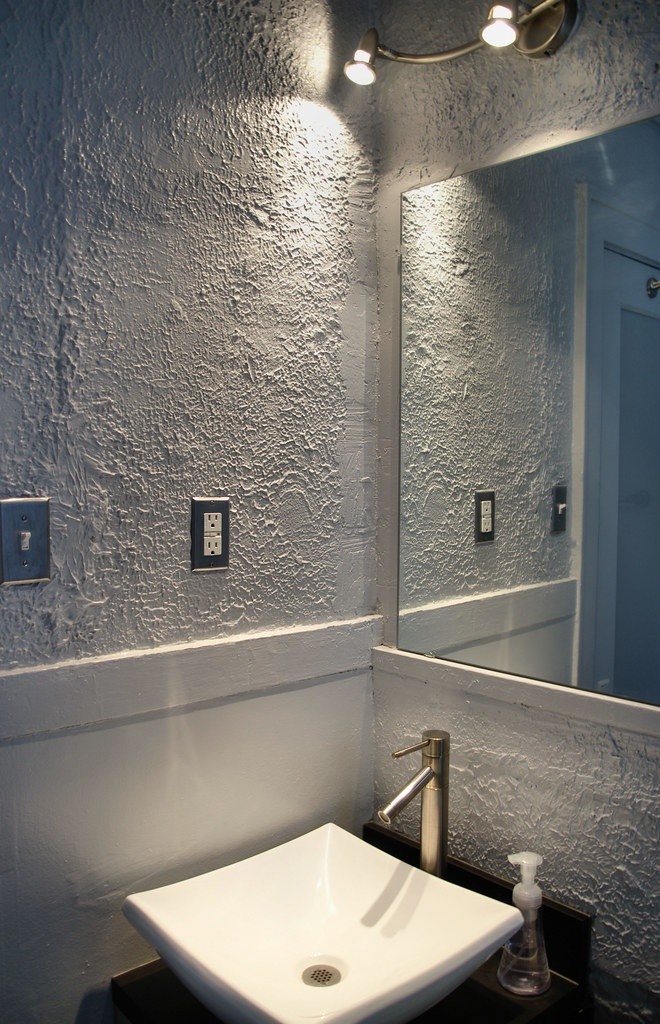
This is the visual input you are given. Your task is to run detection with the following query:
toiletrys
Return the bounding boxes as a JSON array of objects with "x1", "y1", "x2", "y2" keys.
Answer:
[{"x1": 496, "y1": 851, "x2": 550, "y2": 996}]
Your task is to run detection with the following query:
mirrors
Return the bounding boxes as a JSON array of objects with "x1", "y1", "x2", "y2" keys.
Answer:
[{"x1": 397, "y1": 118, "x2": 660, "y2": 707}]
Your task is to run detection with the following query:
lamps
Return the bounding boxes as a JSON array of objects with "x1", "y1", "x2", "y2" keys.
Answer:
[{"x1": 344, "y1": 0, "x2": 579, "y2": 86}]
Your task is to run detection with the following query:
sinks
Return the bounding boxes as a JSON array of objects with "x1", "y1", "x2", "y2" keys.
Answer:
[{"x1": 119, "y1": 822, "x2": 524, "y2": 1024}]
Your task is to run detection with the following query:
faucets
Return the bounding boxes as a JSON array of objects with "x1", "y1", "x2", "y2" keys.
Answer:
[{"x1": 377, "y1": 729, "x2": 452, "y2": 874}]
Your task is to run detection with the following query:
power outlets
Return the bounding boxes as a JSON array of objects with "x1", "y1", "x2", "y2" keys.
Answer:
[
  {"x1": 475, "y1": 489, "x2": 497, "y2": 545},
  {"x1": 191, "y1": 498, "x2": 229, "y2": 572}
]
